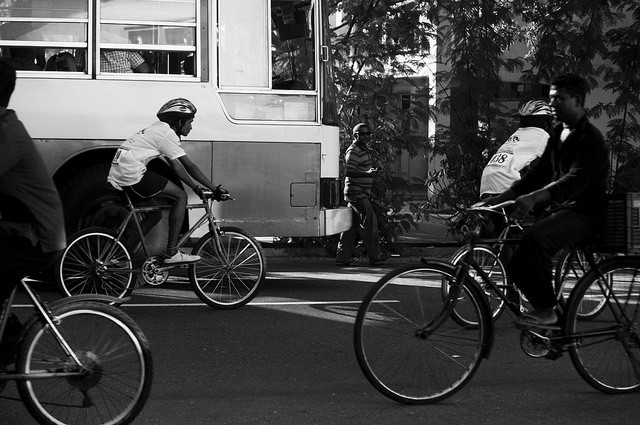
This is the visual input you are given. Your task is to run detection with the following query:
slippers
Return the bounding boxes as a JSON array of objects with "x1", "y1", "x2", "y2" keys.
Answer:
[{"x1": 509, "y1": 312, "x2": 563, "y2": 331}]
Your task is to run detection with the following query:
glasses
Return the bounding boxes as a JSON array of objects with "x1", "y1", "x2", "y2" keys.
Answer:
[{"x1": 355, "y1": 131, "x2": 371, "y2": 136}]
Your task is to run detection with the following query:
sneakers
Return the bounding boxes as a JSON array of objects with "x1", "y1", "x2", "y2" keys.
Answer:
[
  {"x1": 337, "y1": 259, "x2": 358, "y2": 267},
  {"x1": 162, "y1": 248, "x2": 201, "y2": 265},
  {"x1": 369, "y1": 251, "x2": 392, "y2": 267},
  {"x1": 111, "y1": 253, "x2": 130, "y2": 270}
]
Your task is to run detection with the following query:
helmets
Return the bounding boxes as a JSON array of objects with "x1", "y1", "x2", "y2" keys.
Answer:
[
  {"x1": 157, "y1": 98, "x2": 197, "y2": 116},
  {"x1": 519, "y1": 98, "x2": 552, "y2": 119}
]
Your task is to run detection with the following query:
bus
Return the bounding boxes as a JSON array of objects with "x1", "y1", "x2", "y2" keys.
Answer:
[{"x1": 0, "y1": 0, "x2": 355, "y2": 291}]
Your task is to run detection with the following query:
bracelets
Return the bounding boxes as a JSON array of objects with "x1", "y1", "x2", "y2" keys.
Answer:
[{"x1": 192, "y1": 184, "x2": 201, "y2": 192}]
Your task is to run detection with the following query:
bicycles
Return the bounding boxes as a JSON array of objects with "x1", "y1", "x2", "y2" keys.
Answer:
[
  {"x1": 441, "y1": 206, "x2": 613, "y2": 330},
  {"x1": 0, "y1": 259, "x2": 154, "y2": 425},
  {"x1": 351, "y1": 200, "x2": 639, "y2": 406},
  {"x1": 55, "y1": 182, "x2": 268, "y2": 311}
]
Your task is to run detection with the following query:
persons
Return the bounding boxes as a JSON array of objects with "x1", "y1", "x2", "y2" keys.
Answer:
[
  {"x1": 486, "y1": 72, "x2": 610, "y2": 329},
  {"x1": 1, "y1": 2, "x2": 46, "y2": 71},
  {"x1": 336, "y1": 122, "x2": 385, "y2": 268},
  {"x1": 108, "y1": 98, "x2": 228, "y2": 264},
  {"x1": 75, "y1": 29, "x2": 151, "y2": 73},
  {"x1": 54, "y1": 52, "x2": 76, "y2": 72},
  {"x1": 480, "y1": 99, "x2": 562, "y2": 221},
  {"x1": 0, "y1": 59, "x2": 68, "y2": 371}
]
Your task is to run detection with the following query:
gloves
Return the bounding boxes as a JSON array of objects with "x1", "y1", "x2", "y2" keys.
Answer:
[
  {"x1": 213, "y1": 183, "x2": 230, "y2": 202},
  {"x1": 194, "y1": 185, "x2": 211, "y2": 198}
]
